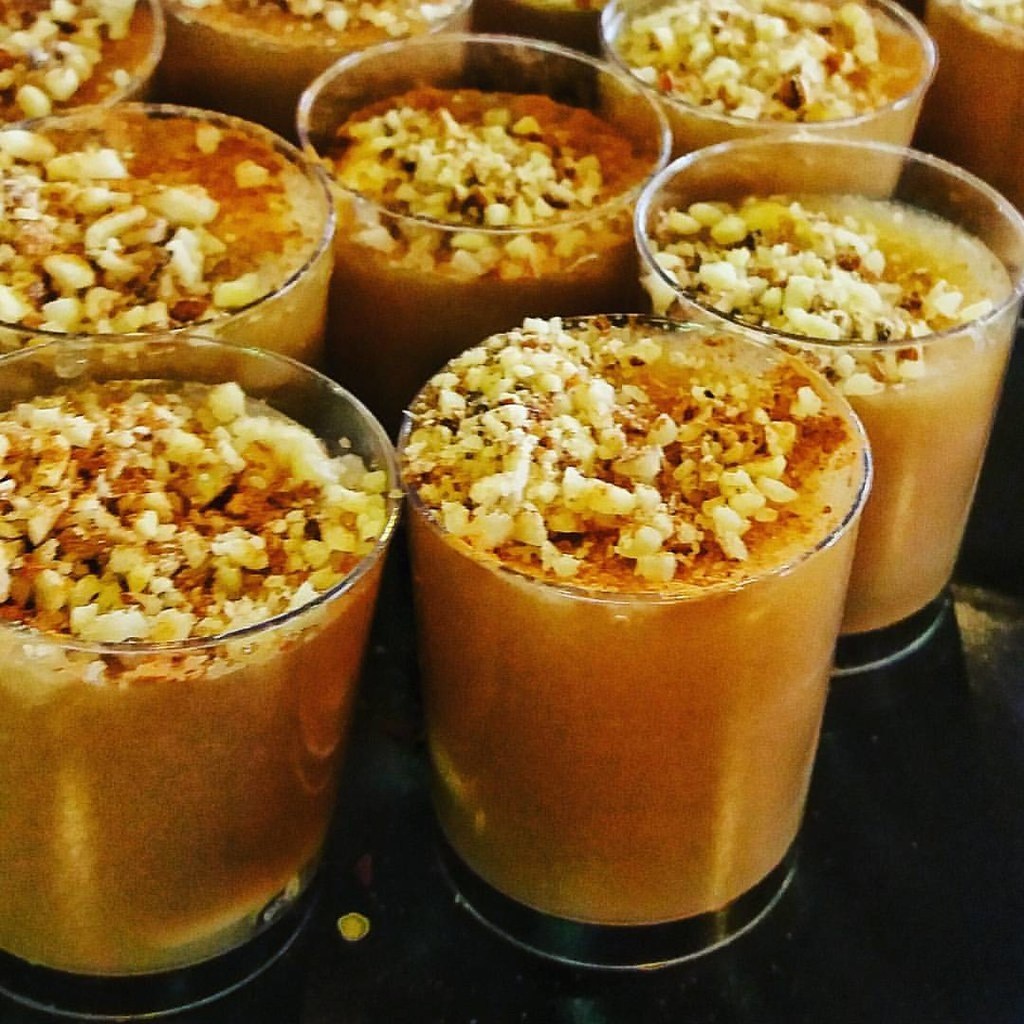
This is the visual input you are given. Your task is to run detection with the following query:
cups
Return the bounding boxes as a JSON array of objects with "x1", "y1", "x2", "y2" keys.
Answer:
[
  {"x1": 296, "y1": 28, "x2": 671, "y2": 415},
  {"x1": 2, "y1": 0, "x2": 168, "y2": 109},
  {"x1": 596, "y1": 3, "x2": 934, "y2": 197},
  {"x1": 2, "y1": 334, "x2": 399, "y2": 1024},
  {"x1": 5, "y1": 106, "x2": 333, "y2": 425},
  {"x1": 393, "y1": 315, "x2": 871, "y2": 972},
  {"x1": 634, "y1": 137, "x2": 1024, "y2": 672},
  {"x1": 940, "y1": 9, "x2": 1018, "y2": 209},
  {"x1": 174, "y1": 3, "x2": 471, "y2": 131}
]
